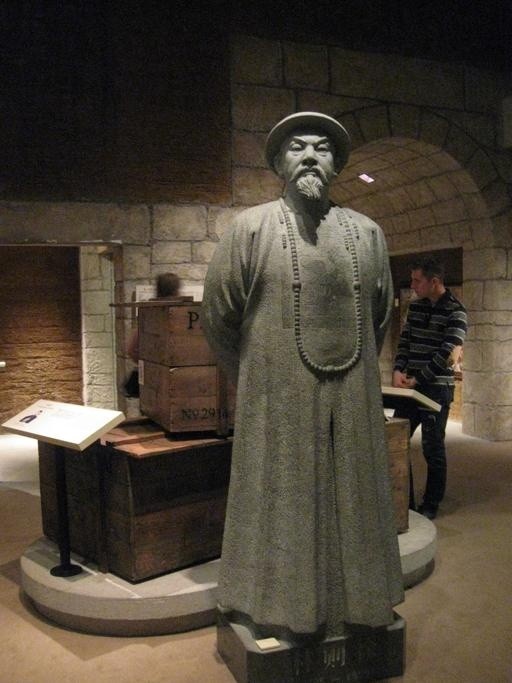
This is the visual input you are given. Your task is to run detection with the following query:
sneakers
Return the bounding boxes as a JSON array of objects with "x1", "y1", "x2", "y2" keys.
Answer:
[{"x1": 415, "y1": 501, "x2": 439, "y2": 520}]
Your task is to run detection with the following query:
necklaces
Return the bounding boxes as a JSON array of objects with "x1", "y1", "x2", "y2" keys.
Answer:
[{"x1": 276, "y1": 193, "x2": 366, "y2": 376}]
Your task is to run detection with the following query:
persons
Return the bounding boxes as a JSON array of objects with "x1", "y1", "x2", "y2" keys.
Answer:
[
  {"x1": 389, "y1": 254, "x2": 466, "y2": 521},
  {"x1": 125, "y1": 271, "x2": 182, "y2": 366},
  {"x1": 19, "y1": 408, "x2": 43, "y2": 424},
  {"x1": 201, "y1": 110, "x2": 406, "y2": 640}
]
